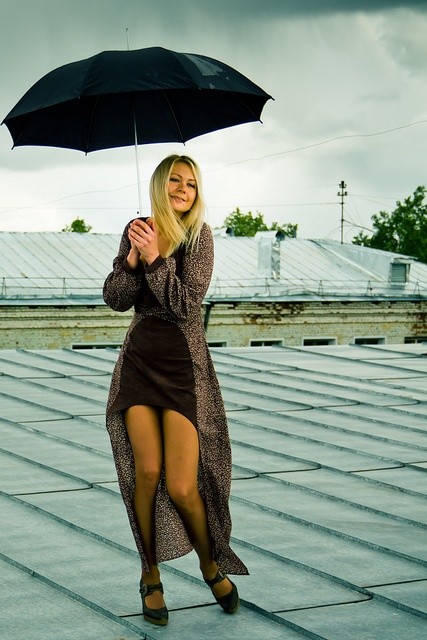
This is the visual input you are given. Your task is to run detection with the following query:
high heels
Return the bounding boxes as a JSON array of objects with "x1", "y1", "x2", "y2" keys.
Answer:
[
  {"x1": 138, "y1": 578, "x2": 168, "y2": 625},
  {"x1": 203, "y1": 570, "x2": 239, "y2": 613}
]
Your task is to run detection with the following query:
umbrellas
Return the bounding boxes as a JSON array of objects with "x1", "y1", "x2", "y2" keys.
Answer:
[{"x1": 1, "y1": 28, "x2": 274, "y2": 219}]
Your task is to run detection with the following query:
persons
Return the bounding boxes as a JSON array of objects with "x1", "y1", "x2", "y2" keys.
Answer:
[{"x1": 102, "y1": 156, "x2": 250, "y2": 625}]
